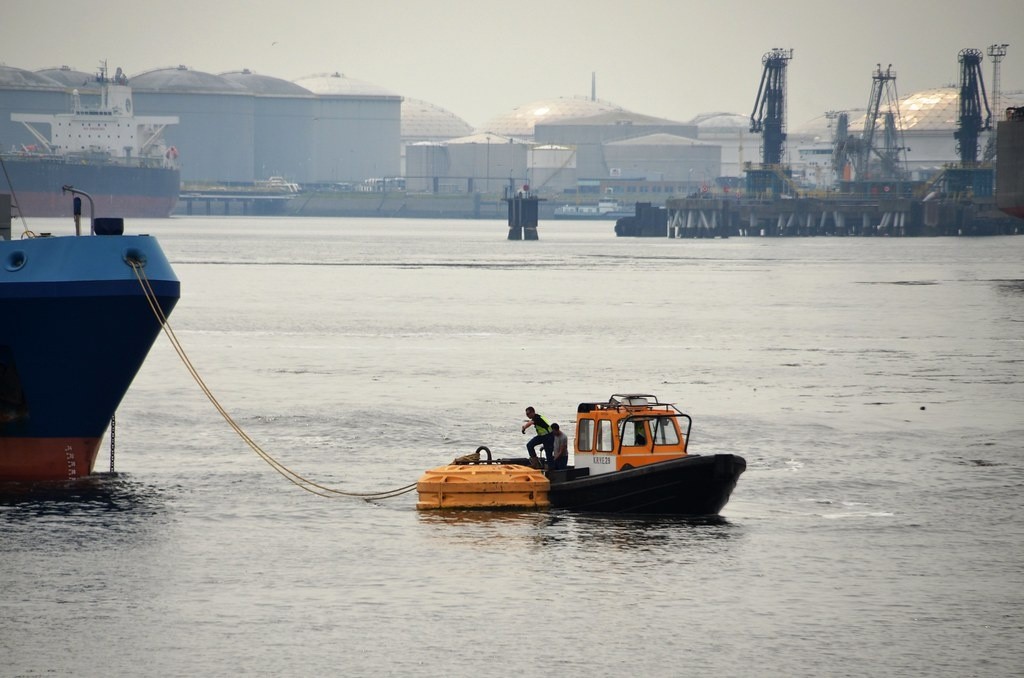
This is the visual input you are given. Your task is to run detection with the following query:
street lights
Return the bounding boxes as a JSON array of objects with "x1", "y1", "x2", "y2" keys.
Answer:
[{"x1": 486, "y1": 137, "x2": 491, "y2": 193}]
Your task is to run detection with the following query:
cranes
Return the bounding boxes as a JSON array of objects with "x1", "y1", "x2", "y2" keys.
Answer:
[
  {"x1": 744, "y1": 49, "x2": 799, "y2": 198},
  {"x1": 950, "y1": 42, "x2": 1008, "y2": 166},
  {"x1": 828, "y1": 62, "x2": 912, "y2": 194}
]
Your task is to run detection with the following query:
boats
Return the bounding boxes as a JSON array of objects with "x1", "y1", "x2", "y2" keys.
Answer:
[
  {"x1": 500, "y1": 394, "x2": 746, "y2": 516},
  {"x1": 0, "y1": 58, "x2": 181, "y2": 221},
  {"x1": 0, "y1": 155, "x2": 182, "y2": 485}
]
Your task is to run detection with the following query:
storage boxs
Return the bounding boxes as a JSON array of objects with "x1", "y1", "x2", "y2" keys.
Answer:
[
  {"x1": 501, "y1": 458, "x2": 529, "y2": 466},
  {"x1": 551, "y1": 469, "x2": 576, "y2": 483}
]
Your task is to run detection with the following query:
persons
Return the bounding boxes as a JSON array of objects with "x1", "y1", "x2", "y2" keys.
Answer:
[
  {"x1": 635, "y1": 427, "x2": 646, "y2": 446},
  {"x1": 550, "y1": 424, "x2": 568, "y2": 469},
  {"x1": 522, "y1": 407, "x2": 554, "y2": 468}
]
